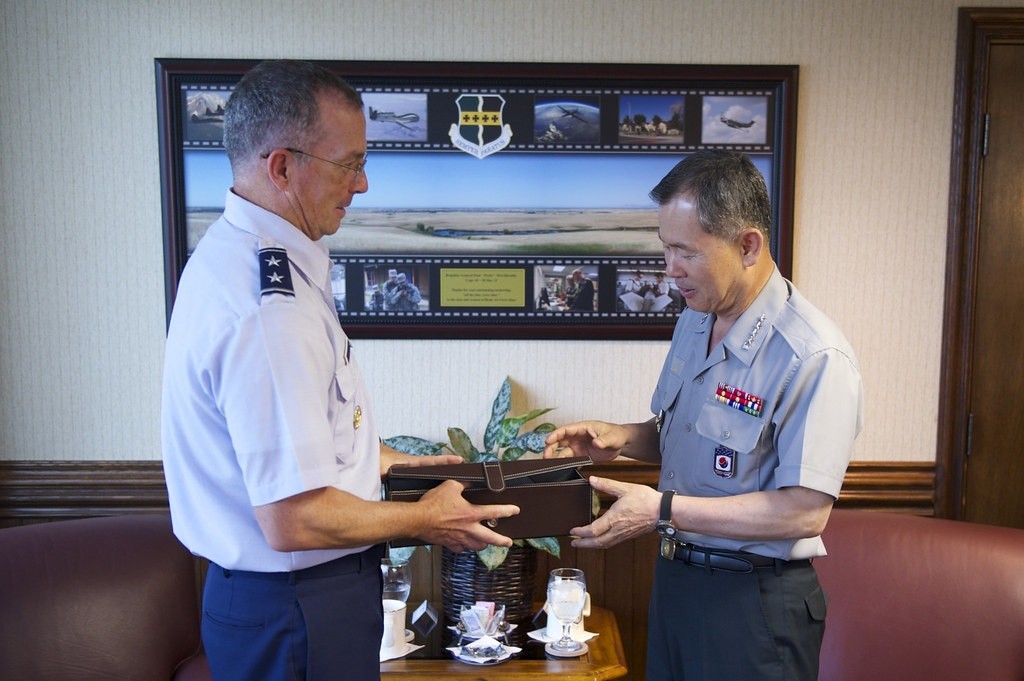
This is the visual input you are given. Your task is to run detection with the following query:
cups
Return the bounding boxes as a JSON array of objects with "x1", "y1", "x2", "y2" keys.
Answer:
[
  {"x1": 545, "y1": 584, "x2": 591, "y2": 641},
  {"x1": 381, "y1": 598, "x2": 409, "y2": 652}
]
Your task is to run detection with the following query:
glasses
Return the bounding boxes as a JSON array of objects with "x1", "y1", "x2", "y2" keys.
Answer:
[{"x1": 259, "y1": 145, "x2": 370, "y2": 181}]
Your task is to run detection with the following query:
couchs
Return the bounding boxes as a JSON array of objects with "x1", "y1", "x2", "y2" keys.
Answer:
[
  {"x1": 0, "y1": 514, "x2": 214, "y2": 681},
  {"x1": 805, "y1": 511, "x2": 1024, "y2": 681}
]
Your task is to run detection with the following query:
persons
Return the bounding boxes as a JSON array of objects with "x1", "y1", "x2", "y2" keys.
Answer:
[
  {"x1": 542, "y1": 148, "x2": 866, "y2": 681},
  {"x1": 618, "y1": 271, "x2": 669, "y2": 311},
  {"x1": 369, "y1": 269, "x2": 421, "y2": 311},
  {"x1": 161, "y1": 58, "x2": 520, "y2": 681},
  {"x1": 547, "y1": 269, "x2": 594, "y2": 311}
]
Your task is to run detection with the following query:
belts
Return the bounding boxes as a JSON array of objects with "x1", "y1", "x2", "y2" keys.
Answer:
[{"x1": 661, "y1": 537, "x2": 776, "y2": 574}]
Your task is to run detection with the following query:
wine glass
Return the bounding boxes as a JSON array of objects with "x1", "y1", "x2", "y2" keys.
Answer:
[
  {"x1": 548, "y1": 568, "x2": 586, "y2": 652},
  {"x1": 380, "y1": 557, "x2": 413, "y2": 637}
]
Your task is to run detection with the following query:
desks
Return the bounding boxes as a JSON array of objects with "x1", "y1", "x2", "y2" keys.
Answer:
[{"x1": 380, "y1": 599, "x2": 628, "y2": 681}]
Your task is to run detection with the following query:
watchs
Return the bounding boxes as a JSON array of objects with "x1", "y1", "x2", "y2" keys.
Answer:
[{"x1": 655, "y1": 489, "x2": 678, "y2": 540}]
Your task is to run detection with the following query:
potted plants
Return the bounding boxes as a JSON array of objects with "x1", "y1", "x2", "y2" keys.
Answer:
[{"x1": 382, "y1": 374, "x2": 561, "y2": 623}]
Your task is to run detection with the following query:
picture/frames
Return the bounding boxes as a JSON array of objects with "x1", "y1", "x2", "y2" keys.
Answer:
[{"x1": 156, "y1": 57, "x2": 801, "y2": 342}]
